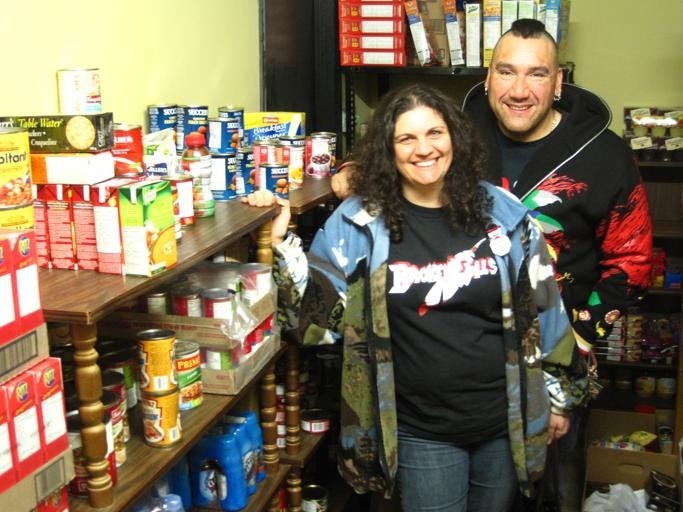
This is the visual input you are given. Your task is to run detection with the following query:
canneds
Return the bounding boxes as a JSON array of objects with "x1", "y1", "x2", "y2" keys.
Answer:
[
  {"x1": 301, "y1": 484, "x2": 329, "y2": 512},
  {"x1": 112, "y1": 103, "x2": 336, "y2": 242},
  {"x1": 50, "y1": 260, "x2": 331, "y2": 511},
  {"x1": 0, "y1": 126, "x2": 35, "y2": 230},
  {"x1": 57, "y1": 68, "x2": 102, "y2": 115}
]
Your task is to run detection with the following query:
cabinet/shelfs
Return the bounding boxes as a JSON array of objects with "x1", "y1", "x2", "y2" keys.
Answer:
[
  {"x1": 578, "y1": 158, "x2": 680, "y2": 411},
  {"x1": 36, "y1": 168, "x2": 343, "y2": 512}
]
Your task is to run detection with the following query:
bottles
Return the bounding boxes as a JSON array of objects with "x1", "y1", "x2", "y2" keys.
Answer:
[{"x1": 133, "y1": 412, "x2": 266, "y2": 512}]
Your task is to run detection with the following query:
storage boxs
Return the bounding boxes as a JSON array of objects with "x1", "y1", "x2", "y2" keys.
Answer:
[{"x1": 582, "y1": 406, "x2": 682, "y2": 499}]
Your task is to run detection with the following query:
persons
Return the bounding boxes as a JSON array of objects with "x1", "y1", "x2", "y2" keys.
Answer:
[
  {"x1": 331, "y1": 17, "x2": 651, "y2": 512},
  {"x1": 239, "y1": 82, "x2": 599, "y2": 511}
]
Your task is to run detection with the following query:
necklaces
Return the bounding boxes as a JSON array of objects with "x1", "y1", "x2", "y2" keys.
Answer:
[{"x1": 549, "y1": 109, "x2": 556, "y2": 132}]
